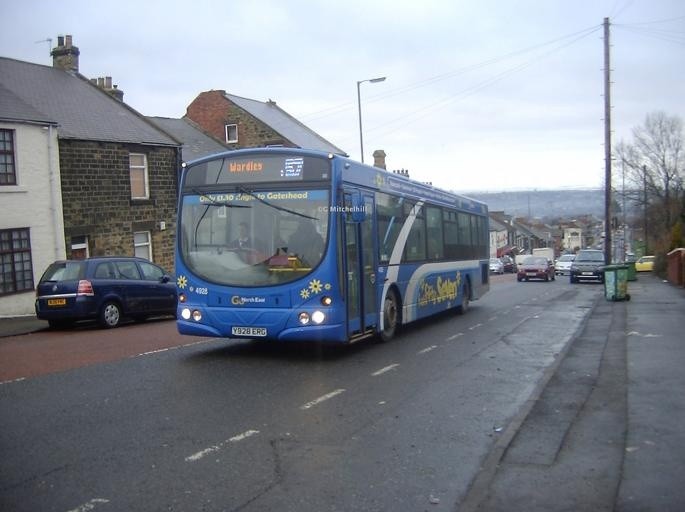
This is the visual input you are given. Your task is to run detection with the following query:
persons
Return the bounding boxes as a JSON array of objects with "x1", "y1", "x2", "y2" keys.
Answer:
[
  {"x1": 288, "y1": 212, "x2": 326, "y2": 268},
  {"x1": 228, "y1": 221, "x2": 265, "y2": 259}
]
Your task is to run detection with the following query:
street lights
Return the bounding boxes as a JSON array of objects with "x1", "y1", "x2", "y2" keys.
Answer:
[{"x1": 357, "y1": 77, "x2": 386, "y2": 164}]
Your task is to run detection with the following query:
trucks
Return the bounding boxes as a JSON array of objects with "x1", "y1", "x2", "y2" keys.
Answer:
[{"x1": 533, "y1": 248, "x2": 555, "y2": 265}]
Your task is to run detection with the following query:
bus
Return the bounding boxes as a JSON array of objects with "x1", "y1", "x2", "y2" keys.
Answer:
[{"x1": 175, "y1": 145, "x2": 490, "y2": 347}]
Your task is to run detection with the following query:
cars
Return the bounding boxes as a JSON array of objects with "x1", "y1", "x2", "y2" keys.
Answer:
[
  {"x1": 517, "y1": 257, "x2": 555, "y2": 281},
  {"x1": 489, "y1": 256, "x2": 516, "y2": 274},
  {"x1": 571, "y1": 250, "x2": 605, "y2": 282},
  {"x1": 635, "y1": 256, "x2": 656, "y2": 272},
  {"x1": 555, "y1": 254, "x2": 577, "y2": 275}
]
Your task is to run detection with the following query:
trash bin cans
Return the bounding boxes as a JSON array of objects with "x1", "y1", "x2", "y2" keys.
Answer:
[
  {"x1": 602, "y1": 265, "x2": 629, "y2": 301},
  {"x1": 624, "y1": 261, "x2": 636, "y2": 280}
]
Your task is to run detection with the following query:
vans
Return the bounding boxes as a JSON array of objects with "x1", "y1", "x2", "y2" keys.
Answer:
[{"x1": 35, "y1": 257, "x2": 178, "y2": 330}]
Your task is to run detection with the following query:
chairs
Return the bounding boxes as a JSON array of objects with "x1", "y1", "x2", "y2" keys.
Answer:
[
  {"x1": 96, "y1": 269, "x2": 107, "y2": 279},
  {"x1": 121, "y1": 268, "x2": 136, "y2": 280}
]
